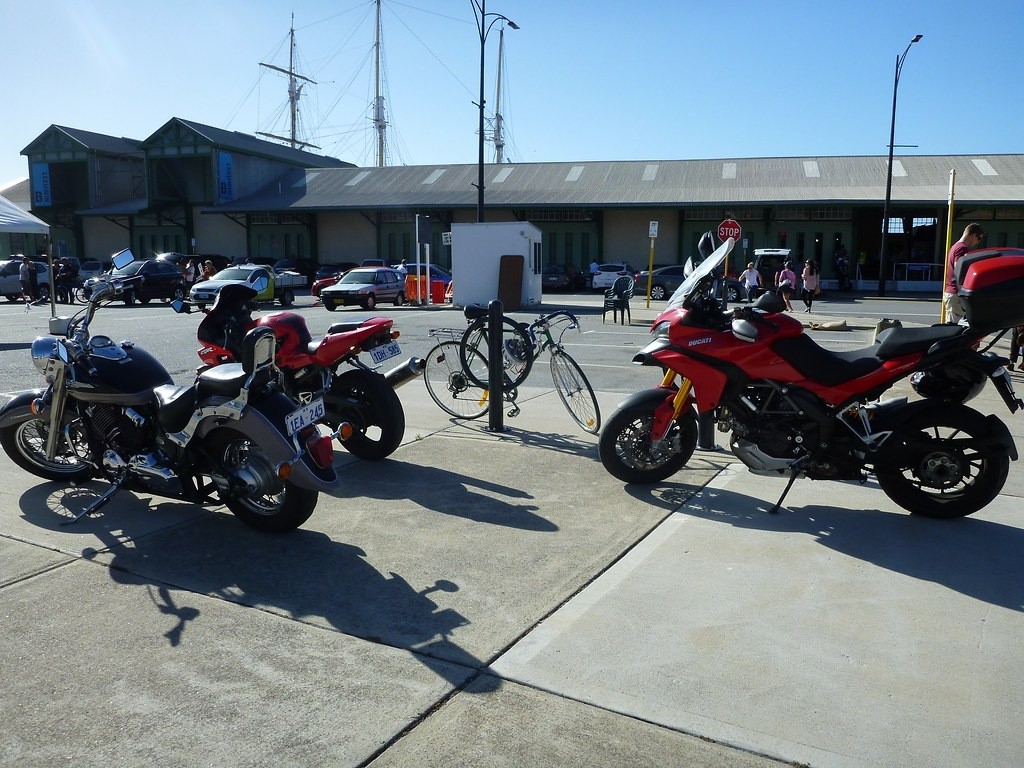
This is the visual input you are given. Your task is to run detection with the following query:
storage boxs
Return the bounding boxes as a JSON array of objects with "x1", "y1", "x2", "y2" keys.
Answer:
[{"x1": 953, "y1": 245, "x2": 1024, "y2": 332}]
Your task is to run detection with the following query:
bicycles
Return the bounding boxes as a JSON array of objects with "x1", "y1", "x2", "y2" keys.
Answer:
[
  {"x1": 75, "y1": 286, "x2": 90, "y2": 303},
  {"x1": 424, "y1": 302, "x2": 601, "y2": 435}
]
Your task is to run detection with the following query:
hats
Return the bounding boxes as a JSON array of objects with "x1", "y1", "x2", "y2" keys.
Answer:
[
  {"x1": 784, "y1": 262, "x2": 793, "y2": 267},
  {"x1": 806, "y1": 259, "x2": 813, "y2": 263}
]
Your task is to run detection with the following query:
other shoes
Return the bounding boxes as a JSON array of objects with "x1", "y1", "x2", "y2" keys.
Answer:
[
  {"x1": 1018, "y1": 362, "x2": 1024, "y2": 370},
  {"x1": 1007, "y1": 364, "x2": 1014, "y2": 371},
  {"x1": 805, "y1": 307, "x2": 811, "y2": 313}
]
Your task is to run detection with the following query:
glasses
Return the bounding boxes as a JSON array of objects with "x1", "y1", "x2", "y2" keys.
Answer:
[{"x1": 977, "y1": 235, "x2": 981, "y2": 241}]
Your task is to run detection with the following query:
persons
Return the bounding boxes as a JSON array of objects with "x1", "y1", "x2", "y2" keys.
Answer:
[
  {"x1": 739, "y1": 262, "x2": 762, "y2": 304},
  {"x1": 396, "y1": 259, "x2": 408, "y2": 274},
  {"x1": 1007, "y1": 325, "x2": 1024, "y2": 371},
  {"x1": 778, "y1": 261, "x2": 796, "y2": 313},
  {"x1": 185, "y1": 259, "x2": 195, "y2": 292},
  {"x1": 245, "y1": 257, "x2": 255, "y2": 266},
  {"x1": 19, "y1": 257, "x2": 30, "y2": 304},
  {"x1": 202, "y1": 260, "x2": 217, "y2": 281},
  {"x1": 800, "y1": 259, "x2": 820, "y2": 314},
  {"x1": 943, "y1": 223, "x2": 983, "y2": 325},
  {"x1": 53, "y1": 259, "x2": 60, "y2": 302},
  {"x1": 589, "y1": 258, "x2": 599, "y2": 289},
  {"x1": 774, "y1": 258, "x2": 787, "y2": 298},
  {"x1": 59, "y1": 257, "x2": 76, "y2": 305},
  {"x1": 27, "y1": 261, "x2": 45, "y2": 305},
  {"x1": 707, "y1": 269, "x2": 719, "y2": 298}
]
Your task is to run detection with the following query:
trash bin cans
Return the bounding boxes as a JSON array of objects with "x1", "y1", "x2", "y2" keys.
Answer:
[{"x1": 405, "y1": 274, "x2": 427, "y2": 304}]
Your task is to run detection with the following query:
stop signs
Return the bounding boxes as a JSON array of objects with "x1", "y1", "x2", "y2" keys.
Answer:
[{"x1": 718, "y1": 220, "x2": 742, "y2": 243}]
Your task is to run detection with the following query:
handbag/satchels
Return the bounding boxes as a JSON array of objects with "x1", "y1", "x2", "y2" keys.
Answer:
[
  {"x1": 778, "y1": 278, "x2": 792, "y2": 291},
  {"x1": 814, "y1": 286, "x2": 821, "y2": 294}
]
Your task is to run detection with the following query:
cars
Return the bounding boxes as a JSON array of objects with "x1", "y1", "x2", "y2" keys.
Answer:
[
  {"x1": 750, "y1": 247, "x2": 798, "y2": 297},
  {"x1": 592, "y1": 261, "x2": 634, "y2": 290},
  {"x1": 60, "y1": 253, "x2": 401, "y2": 288},
  {"x1": 542, "y1": 263, "x2": 587, "y2": 293},
  {"x1": 312, "y1": 266, "x2": 406, "y2": 312},
  {"x1": 634, "y1": 265, "x2": 749, "y2": 303},
  {"x1": 718, "y1": 265, "x2": 738, "y2": 277},
  {"x1": 390, "y1": 264, "x2": 453, "y2": 289}
]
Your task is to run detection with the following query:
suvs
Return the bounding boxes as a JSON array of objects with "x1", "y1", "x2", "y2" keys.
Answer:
[
  {"x1": 0, "y1": 259, "x2": 52, "y2": 303},
  {"x1": 83, "y1": 259, "x2": 185, "y2": 307}
]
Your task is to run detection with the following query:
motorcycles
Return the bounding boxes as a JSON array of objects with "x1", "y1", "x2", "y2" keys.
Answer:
[
  {"x1": 596, "y1": 235, "x2": 1024, "y2": 521},
  {"x1": 168, "y1": 283, "x2": 427, "y2": 461},
  {"x1": 0, "y1": 249, "x2": 354, "y2": 535}
]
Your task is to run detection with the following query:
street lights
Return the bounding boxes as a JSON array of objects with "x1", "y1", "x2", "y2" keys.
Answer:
[
  {"x1": 476, "y1": 12, "x2": 523, "y2": 224},
  {"x1": 878, "y1": 34, "x2": 925, "y2": 297}
]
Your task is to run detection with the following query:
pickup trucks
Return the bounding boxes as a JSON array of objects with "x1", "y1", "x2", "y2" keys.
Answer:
[{"x1": 188, "y1": 264, "x2": 307, "y2": 307}]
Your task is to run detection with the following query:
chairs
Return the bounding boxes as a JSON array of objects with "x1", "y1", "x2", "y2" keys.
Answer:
[{"x1": 602, "y1": 275, "x2": 634, "y2": 326}]
